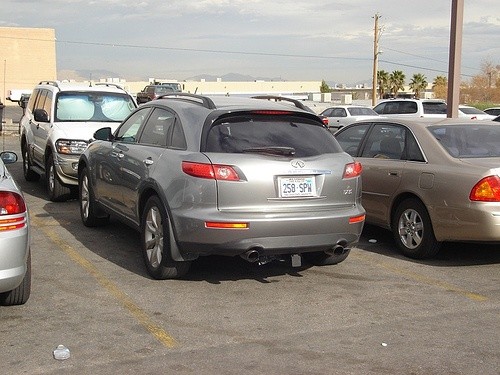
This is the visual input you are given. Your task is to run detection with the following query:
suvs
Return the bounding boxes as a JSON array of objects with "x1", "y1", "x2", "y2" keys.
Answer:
[
  {"x1": 372, "y1": 97, "x2": 475, "y2": 136},
  {"x1": 18, "y1": 79, "x2": 145, "y2": 202},
  {"x1": 137, "y1": 85, "x2": 178, "y2": 105},
  {"x1": 77, "y1": 92, "x2": 367, "y2": 280}
]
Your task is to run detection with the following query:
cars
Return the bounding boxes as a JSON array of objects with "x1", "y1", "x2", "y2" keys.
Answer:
[
  {"x1": 483, "y1": 107, "x2": 500, "y2": 117},
  {"x1": 0, "y1": 150, "x2": 32, "y2": 306},
  {"x1": 332, "y1": 116, "x2": 500, "y2": 260},
  {"x1": 458, "y1": 104, "x2": 496, "y2": 131},
  {"x1": 317, "y1": 105, "x2": 381, "y2": 137}
]
